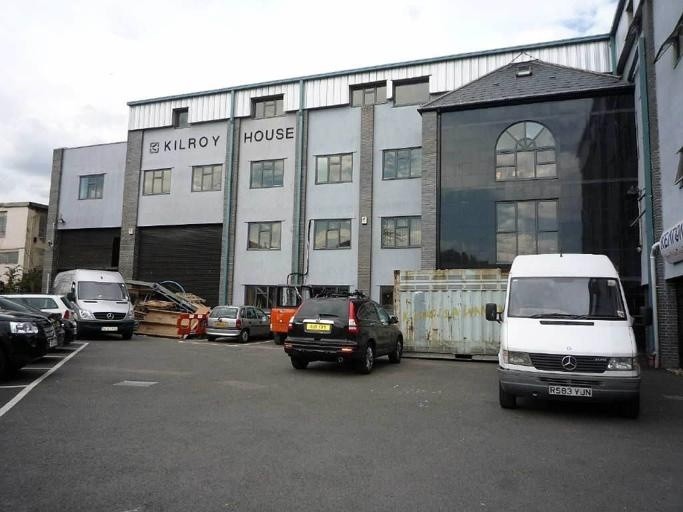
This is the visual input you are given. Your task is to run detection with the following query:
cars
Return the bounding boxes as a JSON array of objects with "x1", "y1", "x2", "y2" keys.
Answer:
[
  {"x1": 204, "y1": 305, "x2": 272, "y2": 343},
  {"x1": 0, "y1": 294, "x2": 78, "y2": 376},
  {"x1": 284, "y1": 289, "x2": 403, "y2": 374}
]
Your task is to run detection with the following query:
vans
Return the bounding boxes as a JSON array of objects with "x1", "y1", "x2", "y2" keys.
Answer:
[
  {"x1": 52, "y1": 269, "x2": 135, "y2": 340},
  {"x1": 485, "y1": 253, "x2": 653, "y2": 418}
]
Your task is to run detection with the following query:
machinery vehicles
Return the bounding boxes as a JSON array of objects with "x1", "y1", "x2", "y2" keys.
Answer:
[{"x1": 270, "y1": 284, "x2": 313, "y2": 345}]
